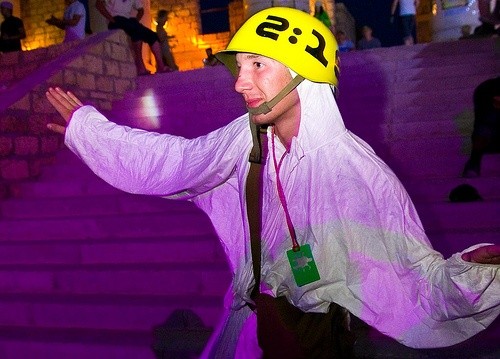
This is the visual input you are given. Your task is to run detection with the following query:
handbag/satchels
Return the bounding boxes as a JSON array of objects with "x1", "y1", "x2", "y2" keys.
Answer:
[{"x1": 249, "y1": 289, "x2": 350, "y2": 359}]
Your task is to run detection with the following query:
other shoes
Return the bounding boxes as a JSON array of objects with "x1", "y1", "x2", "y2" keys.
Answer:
[
  {"x1": 463, "y1": 154, "x2": 481, "y2": 178},
  {"x1": 133, "y1": 63, "x2": 175, "y2": 75}
]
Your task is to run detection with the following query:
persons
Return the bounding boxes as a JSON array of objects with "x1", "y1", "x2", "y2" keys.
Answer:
[
  {"x1": 0, "y1": 2, "x2": 27, "y2": 53},
  {"x1": 95, "y1": 0, "x2": 224, "y2": 76},
  {"x1": 311, "y1": 0, "x2": 500, "y2": 181},
  {"x1": 46, "y1": 0, "x2": 86, "y2": 44},
  {"x1": 45, "y1": 10, "x2": 500, "y2": 359}
]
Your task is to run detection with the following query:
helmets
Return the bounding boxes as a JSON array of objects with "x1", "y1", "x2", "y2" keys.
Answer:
[{"x1": 212, "y1": 6, "x2": 342, "y2": 95}]
